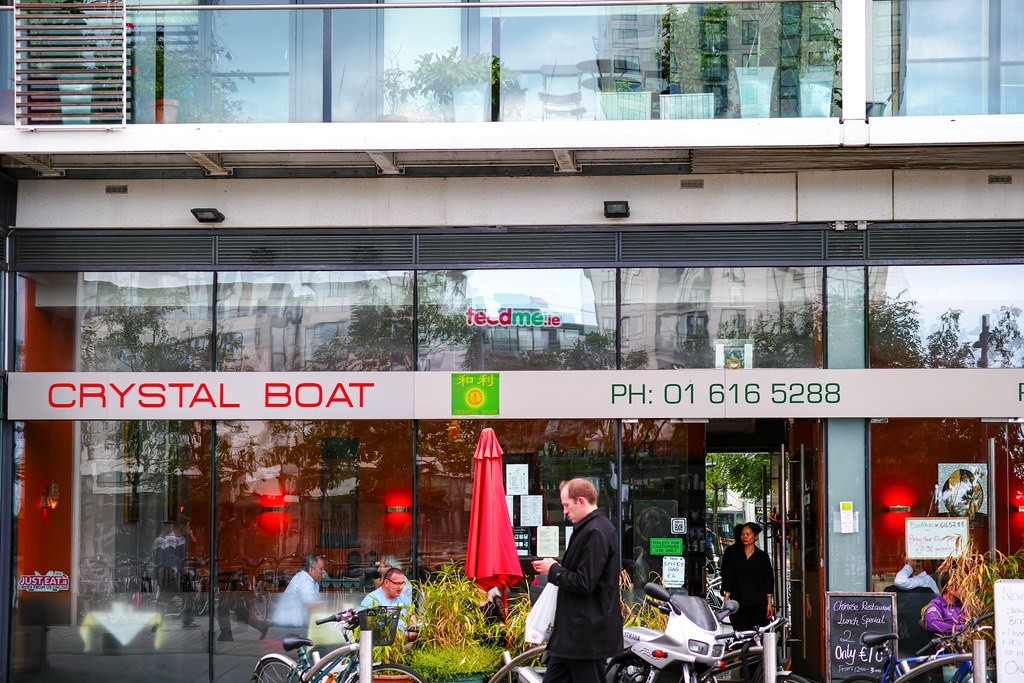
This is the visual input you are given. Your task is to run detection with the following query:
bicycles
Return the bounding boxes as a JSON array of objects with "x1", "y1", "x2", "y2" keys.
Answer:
[
  {"x1": 840, "y1": 611, "x2": 994, "y2": 683},
  {"x1": 250, "y1": 606, "x2": 427, "y2": 683},
  {"x1": 701, "y1": 615, "x2": 810, "y2": 683},
  {"x1": 79, "y1": 557, "x2": 269, "y2": 621}
]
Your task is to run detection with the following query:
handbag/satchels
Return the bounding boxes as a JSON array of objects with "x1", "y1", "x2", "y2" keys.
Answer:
[{"x1": 524, "y1": 582, "x2": 562, "y2": 644}]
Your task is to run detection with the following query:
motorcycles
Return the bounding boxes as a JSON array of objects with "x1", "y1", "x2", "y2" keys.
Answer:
[{"x1": 517, "y1": 581, "x2": 740, "y2": 683}]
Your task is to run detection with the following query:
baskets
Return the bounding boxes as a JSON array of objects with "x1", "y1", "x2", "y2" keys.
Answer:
[{"x1": 357, "y1": 605, "x2": 402, "y2": 645}]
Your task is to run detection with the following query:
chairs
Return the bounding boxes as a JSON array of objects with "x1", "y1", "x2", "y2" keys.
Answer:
[{"x1": 539, "y1": 65, "x2": 584, "y2": 120}]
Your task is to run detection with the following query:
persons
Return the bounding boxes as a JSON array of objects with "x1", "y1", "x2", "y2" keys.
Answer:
[
  {"x1": 360, "y1": 554, "x2": 421, "y2": 637},
  {"x1": 151, "y1": 500, "x2": 198, "y2": 630},
  {"x1": 270, "y1": 554, "x2": 327, "y2": 630},
  {"x1": 214, "y1": 499, "x2": 269, "y2": 641},
  {"x1": 705, "y1": 525, "x2": 718, "y2": 564},
  {"x1": 530, "y1": 478, "x2": 627, "y2": 683},
  {"x1": 895, "y1": 550, "x2": 941, "y2": 597},
  {"x1": 721, "y1": 522, "x2": 781, "y2": 677},
  {"x1": 919, "y1": 574, "x2": 977, "y2": 660}
]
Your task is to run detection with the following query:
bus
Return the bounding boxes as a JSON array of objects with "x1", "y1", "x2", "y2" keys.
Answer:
[{"x1": 706, "y1": 507, "x2": 772, "y2": 554}]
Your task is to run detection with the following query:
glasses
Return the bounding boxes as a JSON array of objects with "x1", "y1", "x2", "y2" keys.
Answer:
[{"x1": 382, "y1": 577, "x2": 405, "y2": 587}]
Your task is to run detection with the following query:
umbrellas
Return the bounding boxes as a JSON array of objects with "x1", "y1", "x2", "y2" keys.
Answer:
[{"x1": 463, "y1": 426, "x2": 522, "y2": 648}]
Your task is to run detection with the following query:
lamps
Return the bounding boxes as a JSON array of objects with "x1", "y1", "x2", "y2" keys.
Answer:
[
  {"x1": 604, "y1": 200, "x2": 630, "y2": 217},
  {"x1": 191, "y1": 208, "x2": 225, "y2": 222}
]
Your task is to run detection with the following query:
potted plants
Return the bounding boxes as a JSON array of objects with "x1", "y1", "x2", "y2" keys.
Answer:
[
  {"x1": 374, "y1": 68, "x2": 415, "y2": 121},
  {"x1": 127, "y1": 32, "x2": 191, "y2": 122},
  {"x1": 408, "y1": 45, "x2": 528, "y2": 122},
  {"x1": 596, "y1": 0, "x2": 843, "y2": 120}
]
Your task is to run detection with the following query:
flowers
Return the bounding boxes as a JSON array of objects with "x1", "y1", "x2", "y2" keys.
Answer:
[{"x1": 95, "y1": 23, "x2": 140, "y2": 123}]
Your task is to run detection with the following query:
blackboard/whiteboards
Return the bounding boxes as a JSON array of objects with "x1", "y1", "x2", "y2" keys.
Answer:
[
  {"x1": 994, "y1": 578, "x2": 1024, "y2": 682},
  {"x1": 904, "y1": 516, "x2": 970, "y2": 560},
  {"x1": 825, "y1": 591, "x2": 898, "y2": 683}
]
